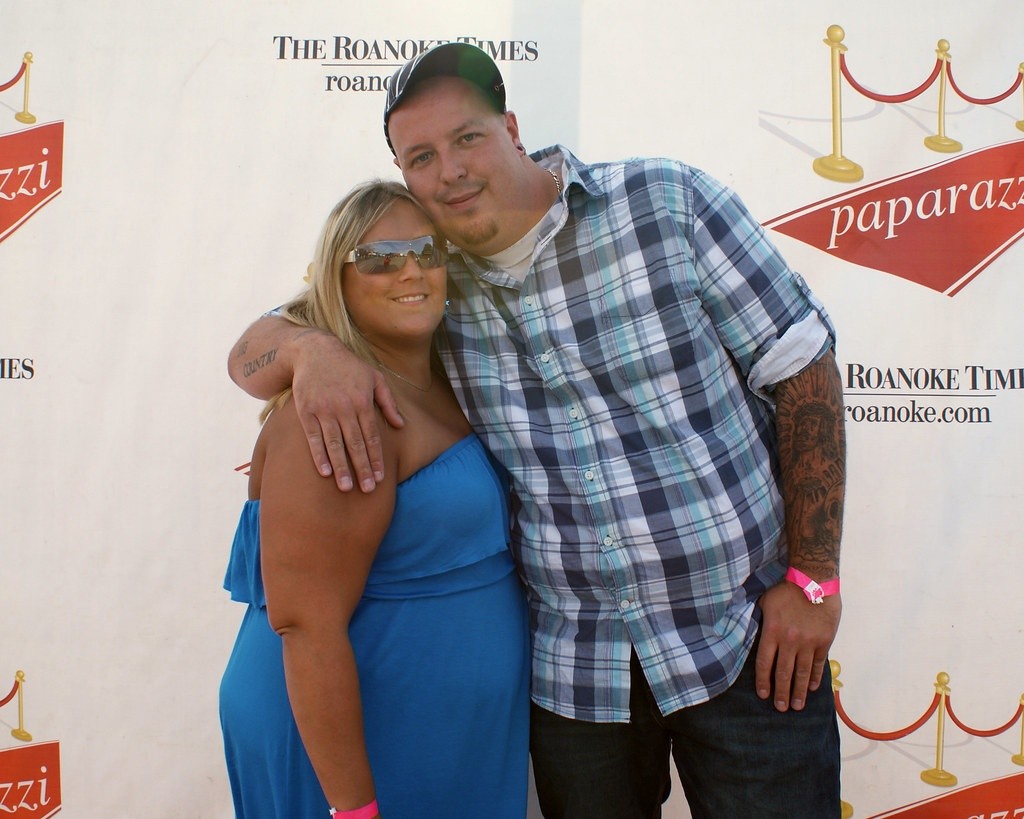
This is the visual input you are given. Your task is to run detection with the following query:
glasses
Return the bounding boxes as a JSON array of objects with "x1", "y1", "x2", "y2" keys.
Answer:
[{"x1": 344, "y1": 234, "x2": 449, "y2": 274}]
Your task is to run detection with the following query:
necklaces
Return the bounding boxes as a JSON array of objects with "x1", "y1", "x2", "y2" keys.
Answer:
[
  {"x1": 376, "y1": 361, "x2": 433, "y2": 392},
  {"x1": 544, "y1": 167, "x2": 562, "y2": 193}
]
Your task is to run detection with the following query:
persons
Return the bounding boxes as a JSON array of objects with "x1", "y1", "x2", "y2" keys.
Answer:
[
  {"x1": 218, "y1": 180, "x2": 533, "y2": 819},
  {"x1": 228, "y1": 43, "x2": 850, "y2": 819}
]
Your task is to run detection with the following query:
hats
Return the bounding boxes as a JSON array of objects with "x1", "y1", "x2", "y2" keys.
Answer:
[{"x1": 384, "y1": 43, "x2": 505, "y2": 159}]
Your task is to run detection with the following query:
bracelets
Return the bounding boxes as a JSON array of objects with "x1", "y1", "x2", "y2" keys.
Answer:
[
  {"x1": 785, "y1": 567, "x2": 840, "y2": 605},
  {"x1": 328, "y1": 799, "x2": 379, "y2": 819}
]
